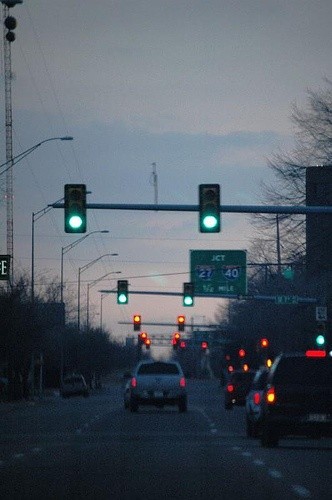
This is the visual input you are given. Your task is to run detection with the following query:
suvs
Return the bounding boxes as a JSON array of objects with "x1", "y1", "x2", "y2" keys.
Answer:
[
  {"x1": 60, "y1": 375, "x2": 89, "y2": 398},
  {"x1": 258, "y1": 348, "x2": 332, "y2": 447},
  {"x1": 124, "y1": 359, "x2": 188, "y2": 413},
  {"x1": 224, "y1": 370, "x2": 263, "y2": 411}
]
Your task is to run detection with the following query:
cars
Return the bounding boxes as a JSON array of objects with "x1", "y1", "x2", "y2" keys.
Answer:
[{"x1": 246, "y1": 365, "x2": 273, "y2": 437}]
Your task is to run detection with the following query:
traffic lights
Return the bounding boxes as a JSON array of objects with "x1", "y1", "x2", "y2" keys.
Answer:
[
  {"x1": 145, "y1": 340, "x2": 151, "y2": 349},
  {"x1": 198, "y1": 182, "x2": 222, "y2": 234},
  {"x1": 64, "y1": 184, "x2": 87, "y2": 234},
  {"x1": 175, "y1": 334, "x2": 181, "y2": 345},
  {"x1": 141, "y1": 332, "x2": 147, "y2": 344},
  {"x1": 173, "y1": 339, "x2": 178, "y2": 350},
  {"x1": 314, "y1": 323, "x2": 328, "y2": 347},
  {"x1": 178, "y1": 316, "x2": 186, "y2": 332},
  {"x1": 133, "y1": 315, "x2": 141, "y2": 331},
  {"x1": 201, "y1": 341, "x2": 207, "y2": 353}
]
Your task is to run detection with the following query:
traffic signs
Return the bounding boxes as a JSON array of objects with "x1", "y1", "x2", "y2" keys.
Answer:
[{"x1": 188, "y1": 249, "x2": 248, "y2": 296}]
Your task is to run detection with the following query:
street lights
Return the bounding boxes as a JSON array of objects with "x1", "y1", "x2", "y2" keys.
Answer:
[
  {"x1": 183, "y1": 282, "x2": 194, "y2": 307},
  {"x1": 117, "y1": 279, "x2": 128, "y2": 305}
]
